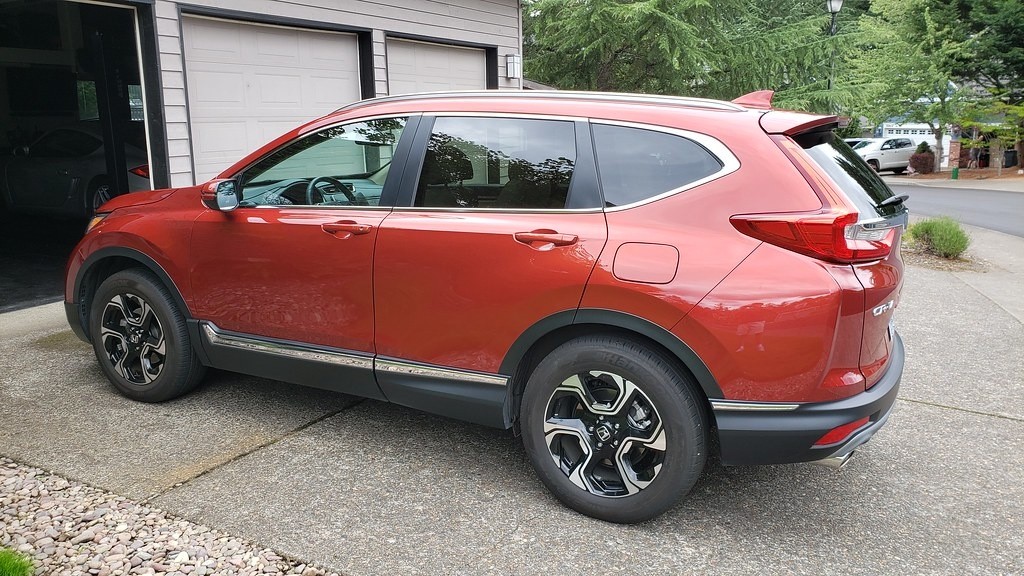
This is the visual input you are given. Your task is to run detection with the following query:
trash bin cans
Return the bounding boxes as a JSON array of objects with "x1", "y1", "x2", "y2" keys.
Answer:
[
  {"x1": 952, "y1": 167, "x2": 959, "y2": 179},
  {"x1": 1004, "y1": 150, "x2": 1017, "y2": 167}
]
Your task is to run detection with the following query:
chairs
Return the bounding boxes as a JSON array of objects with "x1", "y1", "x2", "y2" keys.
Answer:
[
  {"x1": 420, "y1": 146, "x2": 478, "y2": 208},
  {"x1": 490, "y1": 149, "x2": 571, "y2": 208}
]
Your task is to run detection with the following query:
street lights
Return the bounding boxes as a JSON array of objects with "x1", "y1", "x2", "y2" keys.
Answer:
[{"x1": 826, "y1": 0, "x2": 844, "y2": 113}]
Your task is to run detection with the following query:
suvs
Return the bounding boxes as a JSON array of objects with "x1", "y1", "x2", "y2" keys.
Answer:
[{"x1": 60, "y1": 87, "x2": 909, "y2": 526}]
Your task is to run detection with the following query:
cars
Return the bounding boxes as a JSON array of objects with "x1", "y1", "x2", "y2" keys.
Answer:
[
  {"x1": 0, "y1": 118, "x2": 148, "y2": 221},
  {"x1": 850, "y1": 135, "x2": 919, "y2": 174}
]
